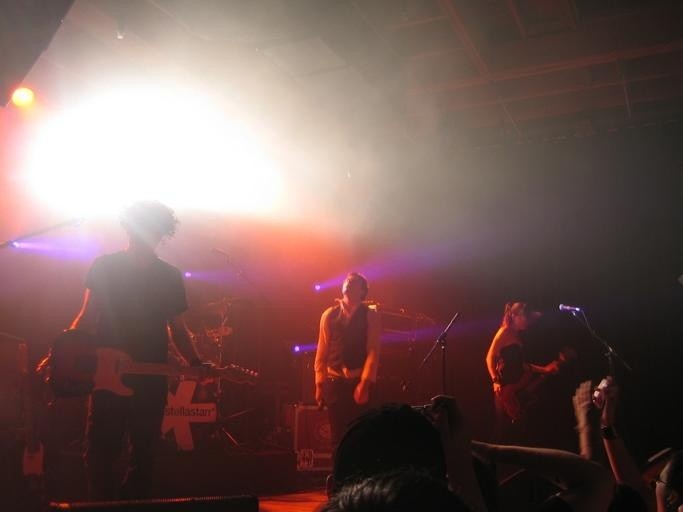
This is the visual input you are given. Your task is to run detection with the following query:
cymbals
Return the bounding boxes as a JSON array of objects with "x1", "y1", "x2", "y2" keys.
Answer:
[{"x1": 205, "y1": 329, "x2": 236, "y2": 338}]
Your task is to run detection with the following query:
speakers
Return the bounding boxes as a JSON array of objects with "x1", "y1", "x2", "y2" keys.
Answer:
[{"x1": 45, "y1": 492, "x2": 260, "y2": 509}]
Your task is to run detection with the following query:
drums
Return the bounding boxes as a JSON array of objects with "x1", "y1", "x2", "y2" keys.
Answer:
[{"x1": 160, "y1": 377, "x2": 222, "y2": 451}]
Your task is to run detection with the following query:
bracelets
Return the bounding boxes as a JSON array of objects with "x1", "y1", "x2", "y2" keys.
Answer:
[{"x1": 189, "y1": 359, "x2": 203, "y2": 367}]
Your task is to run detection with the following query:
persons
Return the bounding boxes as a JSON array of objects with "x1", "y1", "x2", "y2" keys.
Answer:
[
  {"x1": 36, "y1": 202, "x2": 216, "y2": 510},
  {"x1": 314, "y1": 271, "x2": 377, "y2": 453},
  {"x1": 320, "y1": 376, "x2": 682, "y2": 511},
  {"x1": 485, "y1": 302, "x2": 562, "y2": 449}
]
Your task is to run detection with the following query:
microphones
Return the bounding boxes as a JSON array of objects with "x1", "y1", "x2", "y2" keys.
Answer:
[{"x1": 559, "y1": 304, "x2": 581, "y2": 312}]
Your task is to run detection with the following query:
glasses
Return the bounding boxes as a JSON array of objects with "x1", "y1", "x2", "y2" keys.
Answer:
[{"x1": 653, "y1": 472, "x2": 667, "y2": 485}]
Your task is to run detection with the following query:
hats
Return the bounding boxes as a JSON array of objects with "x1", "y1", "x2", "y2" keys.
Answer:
[{"x1": 332, "y1": 402, "x2": 447, "y2": 488}]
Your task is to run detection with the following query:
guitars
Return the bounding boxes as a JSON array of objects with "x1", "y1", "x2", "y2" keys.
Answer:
[
  {"x1": 48, "y1": 327, "x2": 258, "y2": 397},
  {"x1": 499, "y1": 347, "x2": 579, "y2": 419}
]
